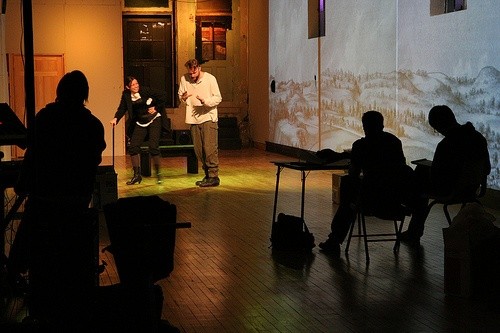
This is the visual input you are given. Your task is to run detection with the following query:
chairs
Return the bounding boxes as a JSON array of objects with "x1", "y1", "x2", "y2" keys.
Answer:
[
  {"x1": 424, "y1": 151, "x2": 484, "y2": 226},
  {"x1": 345, "y1": 163, "x2": 414, "y2": 253}
]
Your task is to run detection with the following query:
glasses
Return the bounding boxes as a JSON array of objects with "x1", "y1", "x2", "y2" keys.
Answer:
[{"x1": 434, "y1": 128, "x2": 439, "y2": 133}]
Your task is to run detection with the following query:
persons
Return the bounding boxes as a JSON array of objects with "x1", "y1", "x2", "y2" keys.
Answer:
[
  {"x1": 5, "y1": 70, "x2": 107, "y2": 283},
  {"x1": 178, "y1": 59, "x2": 223, "y2": 187},
  {"x1": 319, "y1": 110, "x2": 415, "y2": 255},
  {"x1": 111, "y1": 76, "x2": 167, "y2": 186},
  {"x1": 398, "y1": 106, "x2": 491, "y2": 248}
]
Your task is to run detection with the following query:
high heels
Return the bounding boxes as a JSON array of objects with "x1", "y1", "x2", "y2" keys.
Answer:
[
  {"x1": 126, "y1": 166, "x2": 142, "y2": 185},
  {"x1": 151, "y1": 162, "x2": 163, "y2": 184}
]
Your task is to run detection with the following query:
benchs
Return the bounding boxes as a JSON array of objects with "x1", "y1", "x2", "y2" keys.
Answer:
[{"x1": 140, "y1": 144, "x2": 198, "y2": 176}]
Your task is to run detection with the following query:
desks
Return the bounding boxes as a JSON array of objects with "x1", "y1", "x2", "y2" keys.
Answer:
[{"x1": 271, "y1": 161, "x2": 349, "y2": 223}]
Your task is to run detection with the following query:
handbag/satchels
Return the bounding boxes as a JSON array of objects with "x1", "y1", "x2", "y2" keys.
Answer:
[{"x1": 267, "y1": 212, "x2": 316, "y2": 270}]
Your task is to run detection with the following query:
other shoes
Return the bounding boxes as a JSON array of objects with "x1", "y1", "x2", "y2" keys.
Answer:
[
  {"x1": 319, "y1": 238, "x2": 341, "y2": 256},
  {"x1": 200, "y1": 177, "x2": 220, "y2": 187},
  {"x1": 196, "y1": 176, "x2": 209, "y2": 186},
  {"x1": 400, "y1": 230, "x2": 421, "y2": 247}
]
